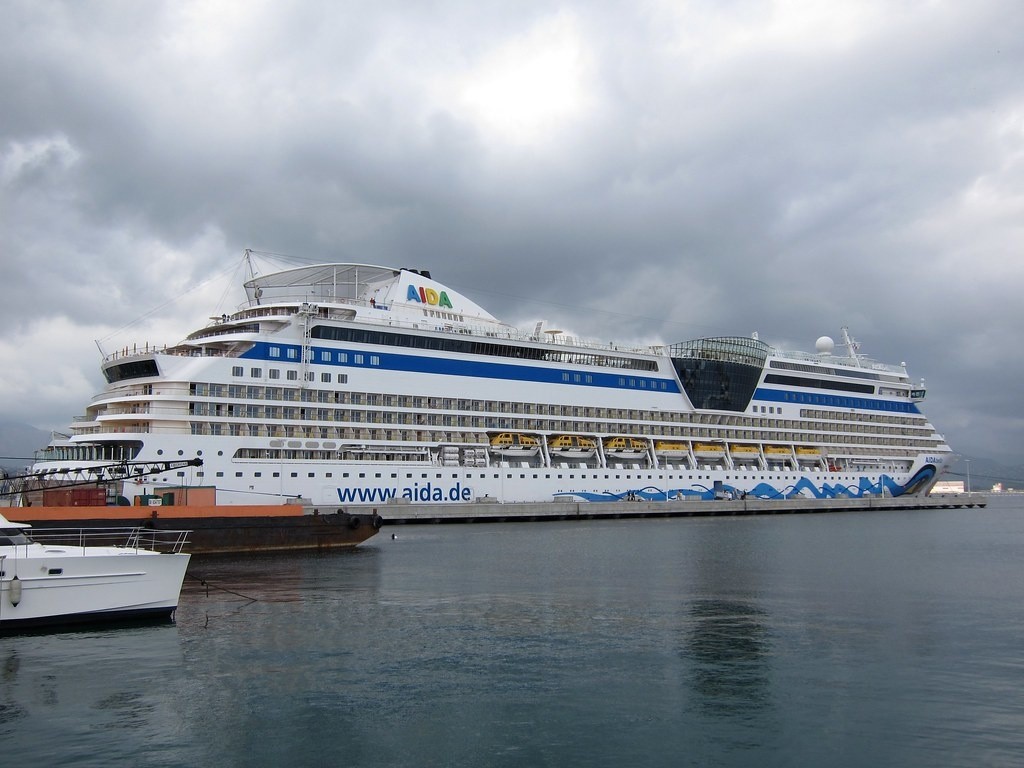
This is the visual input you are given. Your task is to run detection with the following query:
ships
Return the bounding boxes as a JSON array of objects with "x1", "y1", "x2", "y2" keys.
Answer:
[{"x1": 0, "y1": 247, "x2": 955, "y2": 528}]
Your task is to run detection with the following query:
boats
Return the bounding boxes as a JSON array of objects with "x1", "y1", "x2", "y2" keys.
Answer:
[
  {"x1": 603, "y1": 436, "x2": 649, "y2": 459},
  {"x1": 0, "y1": 526, "x2": 194, "y2": 629},
  {"x1": 654, "y1": 442, "x2": 688, "y2": 459},
  {"x1": 490, "y1": 433, "x2": 541, "y2": 457},
  {"x1": 762, "y1": 446, "x2": 794, "y2": 460},
  {"x1": 547, "y1": 435, "x2": 598, "y2": 458},
  {"x1": 729, "y1": 443, "x2": 761, "y2": 461},
  {"x1": 0, "y1": 457, "x2": 384, "y2": 552},
  {"x1": 692, "y1": 444, "x2": 726, "y2": 461},
  {"x1": 795, "y1": 447, "x2": 822, "y2": 461}
]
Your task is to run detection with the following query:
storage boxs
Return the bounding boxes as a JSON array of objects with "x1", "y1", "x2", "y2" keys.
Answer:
[{"x1": 43, "y1": 488, "x2": 106, "y2": 507}]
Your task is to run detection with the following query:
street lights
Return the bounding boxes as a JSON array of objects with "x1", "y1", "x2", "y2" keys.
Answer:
[{"x1": 964, "y1": 460, "x2": 972, "y2": 497}]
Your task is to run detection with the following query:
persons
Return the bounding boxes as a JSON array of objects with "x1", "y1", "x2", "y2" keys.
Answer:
[
  {"x1": 677, "y1": 490, "x2": 682, "y2": 500},
  {"x1": 628, "y1": 491, "x2": 635, "y2": 501},
  {"x1": 370, "y1": 298, "x2": 375, "y2": 307},
  {"x1": 222, "y1": 314, "x2": 230, "y2": 322}
]
